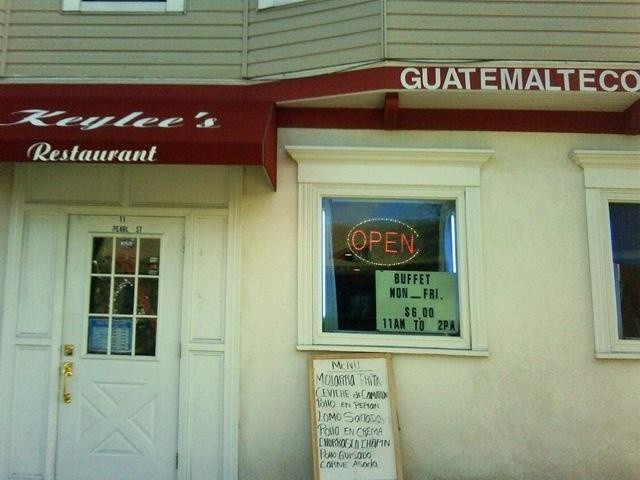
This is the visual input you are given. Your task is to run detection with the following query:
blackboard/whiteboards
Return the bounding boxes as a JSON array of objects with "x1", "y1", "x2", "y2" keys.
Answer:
[{"x1": 308, "y1": 353, "x2": 403, "y2": 480}]
